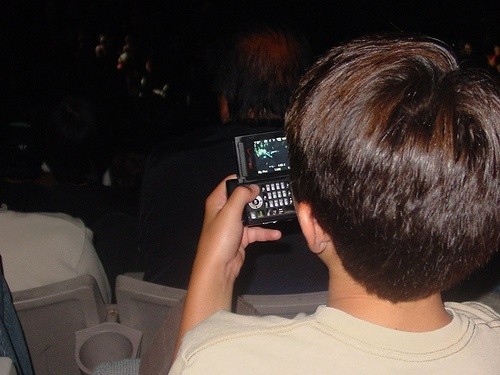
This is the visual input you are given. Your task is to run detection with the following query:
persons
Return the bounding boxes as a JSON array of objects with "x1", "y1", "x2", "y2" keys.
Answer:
[{"x1": 168, "y1": 32, "x2": 500, "y2": 375}]
